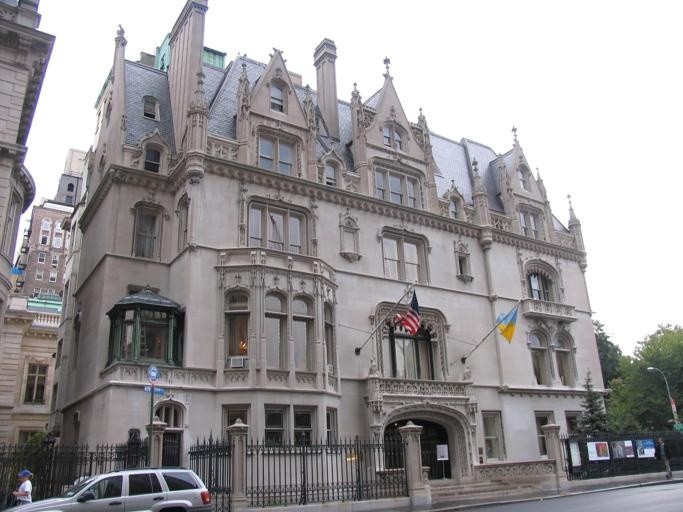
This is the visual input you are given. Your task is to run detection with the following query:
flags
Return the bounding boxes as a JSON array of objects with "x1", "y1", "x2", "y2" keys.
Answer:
[
  {"x1": 492, "y1": 303, "x2": 518, "y2": 344},
  {"x1": 390, "y1": 288, "x2": 421, "y2": 337}
]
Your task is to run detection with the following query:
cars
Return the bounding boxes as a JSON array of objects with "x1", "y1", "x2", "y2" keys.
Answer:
[{"x1": 1, "y1": 468, "x2": 214, "y2": 512}]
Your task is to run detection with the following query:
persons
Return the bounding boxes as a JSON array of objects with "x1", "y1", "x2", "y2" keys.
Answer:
[
  {"x1": 652, "y1": 436, "x2": 674, "y2": 480},
  {"x1": 8, "y1": 468, "x2": 34, "y2": 508}
]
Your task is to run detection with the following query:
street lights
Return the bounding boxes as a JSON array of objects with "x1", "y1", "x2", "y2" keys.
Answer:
[{"x1": 647, "y1": 367, "x2": 680, "y2": 423}]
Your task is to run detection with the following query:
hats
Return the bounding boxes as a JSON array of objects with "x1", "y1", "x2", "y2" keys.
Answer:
[{"x1": 17, "y1": 469, "x2": 31, "y2": 478}]
